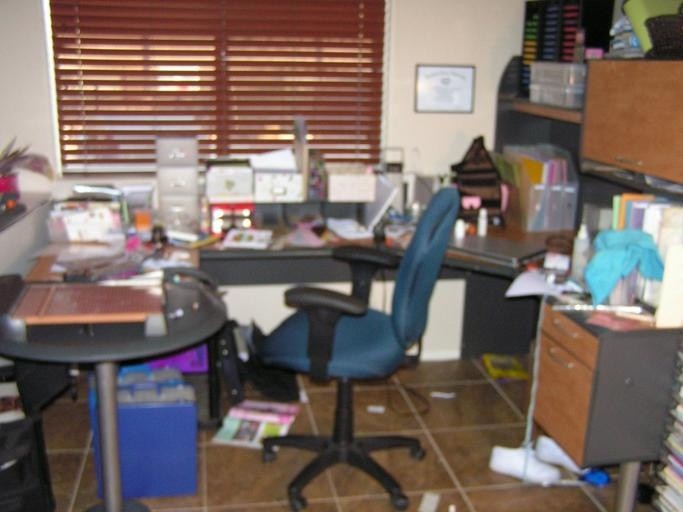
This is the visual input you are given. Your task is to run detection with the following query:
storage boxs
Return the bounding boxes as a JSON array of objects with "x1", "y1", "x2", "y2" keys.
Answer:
[
  {"x1": 152, "y1": 132, "x2": 406, "y2": 250},
  {"x1": 528, "y1": 81, "x2": 584, "y2": 111},
  {"x1": 526, "y1": 60, "x2": 586, "y2": 89}
]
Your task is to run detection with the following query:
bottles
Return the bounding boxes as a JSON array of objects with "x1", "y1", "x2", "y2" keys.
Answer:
[
  {"x1": 455, "y1": 219, "x2": 465, "y2": 239},
  {"x1": 477, "y1": 208, "x2": 489, "y2": 236},
  {"x1": 573, "y1": 222, "x2": 589, "y2": 278}
]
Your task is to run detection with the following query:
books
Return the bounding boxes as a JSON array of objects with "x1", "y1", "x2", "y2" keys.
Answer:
[
  {"x1": 615, "y1": 188, "x2": 683, "y2": 309},
  {"x1": 509, "y1": 148, "x2": 569, "y2": 227},
  {"x1": 13, "y1": 281, "x2": 164, "y2": 326},
  {"x1": 212, "y1": 397, "x2": 300, "y2": 452}
]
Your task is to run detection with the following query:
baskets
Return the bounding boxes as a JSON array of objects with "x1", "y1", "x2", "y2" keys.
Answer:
[{"x1": 644, "y1": 4, "x2": 683, "y2": 57}]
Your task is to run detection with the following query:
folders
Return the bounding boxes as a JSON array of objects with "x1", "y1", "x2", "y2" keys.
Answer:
[{"x1": 497, "y1": 146, "x2": 569, "y2": 229}]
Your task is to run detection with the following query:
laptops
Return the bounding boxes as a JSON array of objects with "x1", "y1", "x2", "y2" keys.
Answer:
[{"x1": 447, "y1": 235, "x2": 547, "y2": 267}]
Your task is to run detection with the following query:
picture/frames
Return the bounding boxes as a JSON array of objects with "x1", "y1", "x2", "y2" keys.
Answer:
[{"x1": 411, "y1": 62, "x2": 478, "y2": 117}]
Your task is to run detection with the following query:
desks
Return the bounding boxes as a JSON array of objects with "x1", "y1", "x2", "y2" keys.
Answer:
[{"x1": 0, "y1": 197, "x2": 602, "y2": 511}]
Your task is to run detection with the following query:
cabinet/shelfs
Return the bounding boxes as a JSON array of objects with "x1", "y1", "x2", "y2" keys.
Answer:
[
  {"x1": 574, "y1": 58, "x2": 683, "y2": 204},
  {"x1": 529, "y1": 287, "x2": 682, "y2": 511}
]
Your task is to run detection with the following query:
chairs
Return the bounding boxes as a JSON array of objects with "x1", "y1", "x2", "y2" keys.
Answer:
[{"x1": 252, "y1": 184, "x2": 463, "y2": 510}]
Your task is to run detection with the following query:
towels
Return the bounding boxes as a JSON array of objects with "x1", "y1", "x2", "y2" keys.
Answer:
[{"x1": 582, "y1": 225, "x2": 663, "y2": 305}]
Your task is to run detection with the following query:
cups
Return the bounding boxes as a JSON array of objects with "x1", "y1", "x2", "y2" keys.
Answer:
[{"x1": 543, "y1": 233, "x2": 572, "y2": 278}]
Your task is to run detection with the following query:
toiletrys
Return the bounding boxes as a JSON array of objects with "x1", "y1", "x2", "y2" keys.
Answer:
[{"x1": 570, "y1": 224, "x2": 590, "y2": 283}]
